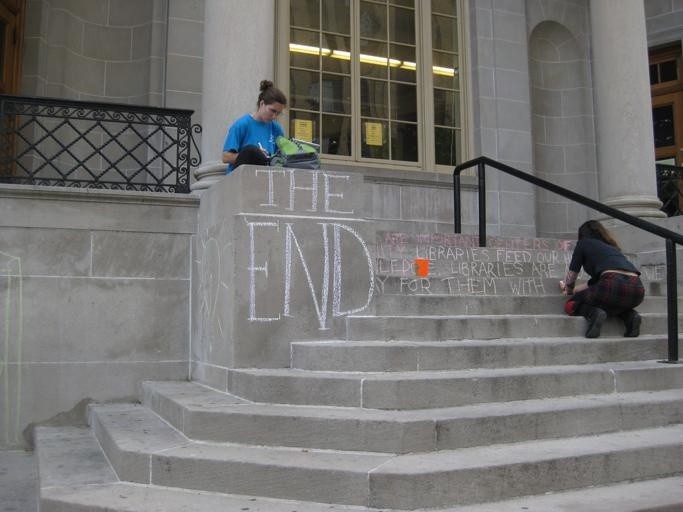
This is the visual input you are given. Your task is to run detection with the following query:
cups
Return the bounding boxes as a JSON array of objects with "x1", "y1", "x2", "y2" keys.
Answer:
[{"x1": 415, "y1": 258, "x2": 429, "y2": 277}]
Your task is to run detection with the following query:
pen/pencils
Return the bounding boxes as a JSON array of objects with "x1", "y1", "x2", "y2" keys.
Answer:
[{"x1": 258, "y1": 141, "x2": 266, "y2": 156}]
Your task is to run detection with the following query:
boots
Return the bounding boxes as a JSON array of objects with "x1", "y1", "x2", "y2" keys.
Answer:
[
  {"x1": 570, "y1": 302, "x2": 607, "y2": 338},
  {"x1": 620, "y1": 308, "x2": 640, "y2": 337}
]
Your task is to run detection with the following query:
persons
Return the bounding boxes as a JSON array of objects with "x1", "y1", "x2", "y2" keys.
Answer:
[
  {"x1": 219, "y1": 78, "x2": 288, "y2": 175},
  {"x1": 558, "y1": 219, "x2": 648, "y2": 339}
]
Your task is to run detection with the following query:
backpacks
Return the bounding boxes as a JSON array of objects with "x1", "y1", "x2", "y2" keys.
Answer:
[{"x1": 274, "y1": 134, "x2": 320, "y2": 169}]
